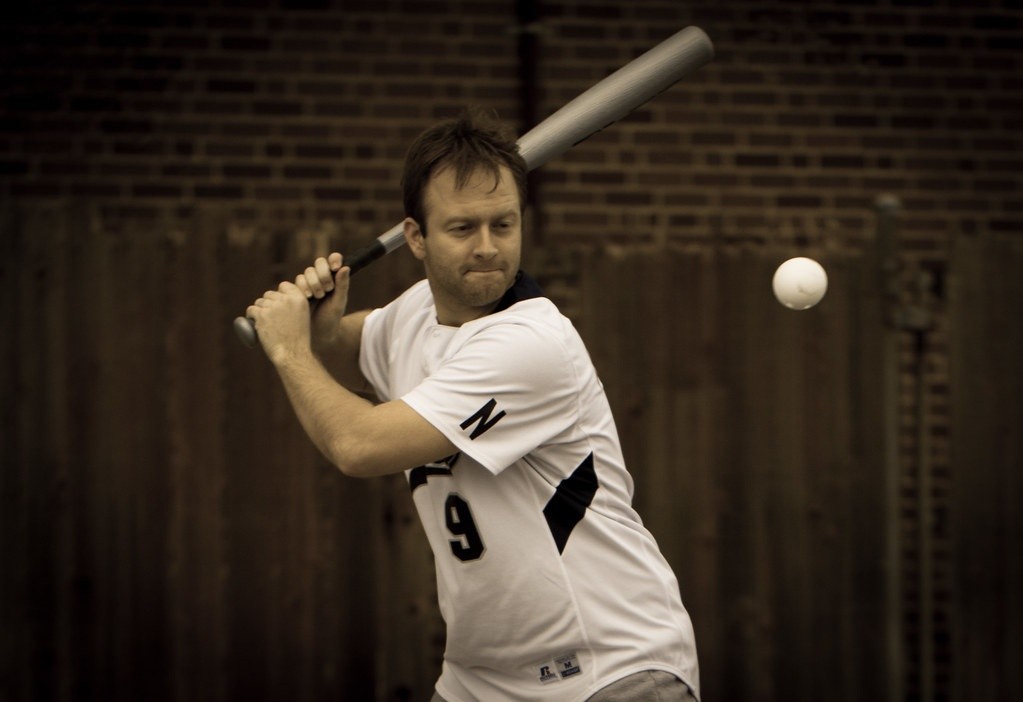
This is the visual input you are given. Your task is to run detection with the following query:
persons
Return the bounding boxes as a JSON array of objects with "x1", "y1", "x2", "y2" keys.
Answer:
[{"x1": 246, "y1": 122, "x2": 700, "y2": 702}]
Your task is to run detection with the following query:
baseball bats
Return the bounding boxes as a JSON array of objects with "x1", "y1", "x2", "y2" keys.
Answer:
[{"x1": 233, "y1": 25, "x2": 719, "y2": 349}]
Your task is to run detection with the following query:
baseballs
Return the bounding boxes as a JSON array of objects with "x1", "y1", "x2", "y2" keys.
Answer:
[{"x1": 774, "y1": 255, "x2": 828, "y2": 312}]
